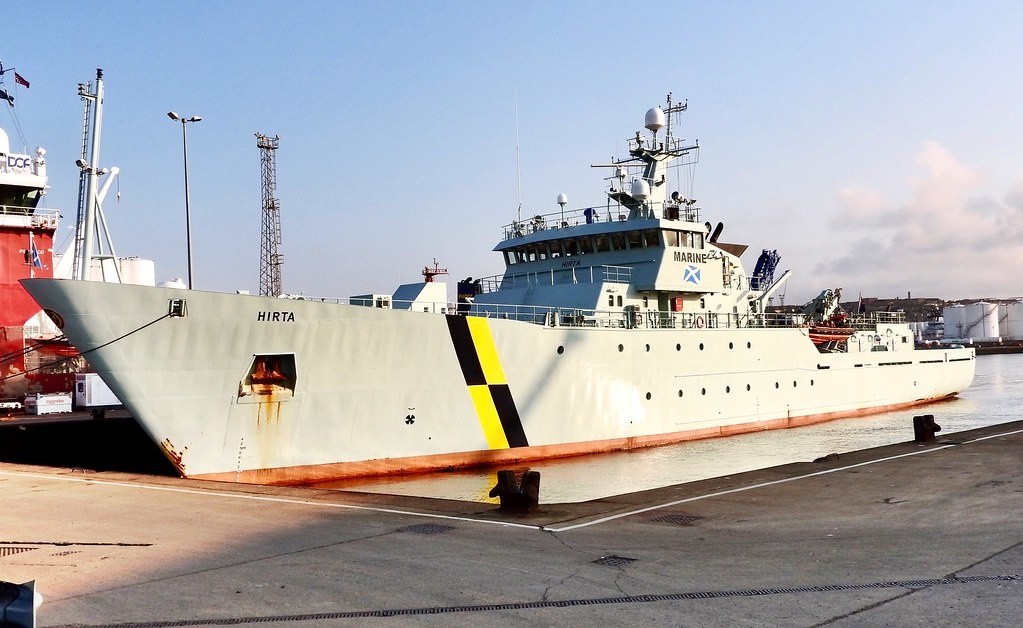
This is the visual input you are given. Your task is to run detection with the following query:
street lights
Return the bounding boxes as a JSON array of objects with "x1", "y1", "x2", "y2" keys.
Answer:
[{"x1": 167, "y1": 111, "x2": 202, "y2": 290}]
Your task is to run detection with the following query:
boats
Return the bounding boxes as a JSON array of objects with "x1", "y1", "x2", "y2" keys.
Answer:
[
  {"x1": 0, "y1": 59, "x2": 186, "y2": 401},
  {"x1": 17, "y1": 91, "x2": 977, "y2": 486}
]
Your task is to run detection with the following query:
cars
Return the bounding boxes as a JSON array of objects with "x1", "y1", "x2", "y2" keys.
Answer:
[{"x1": 0, "y1": 402, "x2": 22, "y2": 410}]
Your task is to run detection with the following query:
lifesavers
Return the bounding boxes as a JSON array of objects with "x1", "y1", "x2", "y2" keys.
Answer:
[{"x1": 696, "y1": 315, "x2": 705, "y2": 329}]
